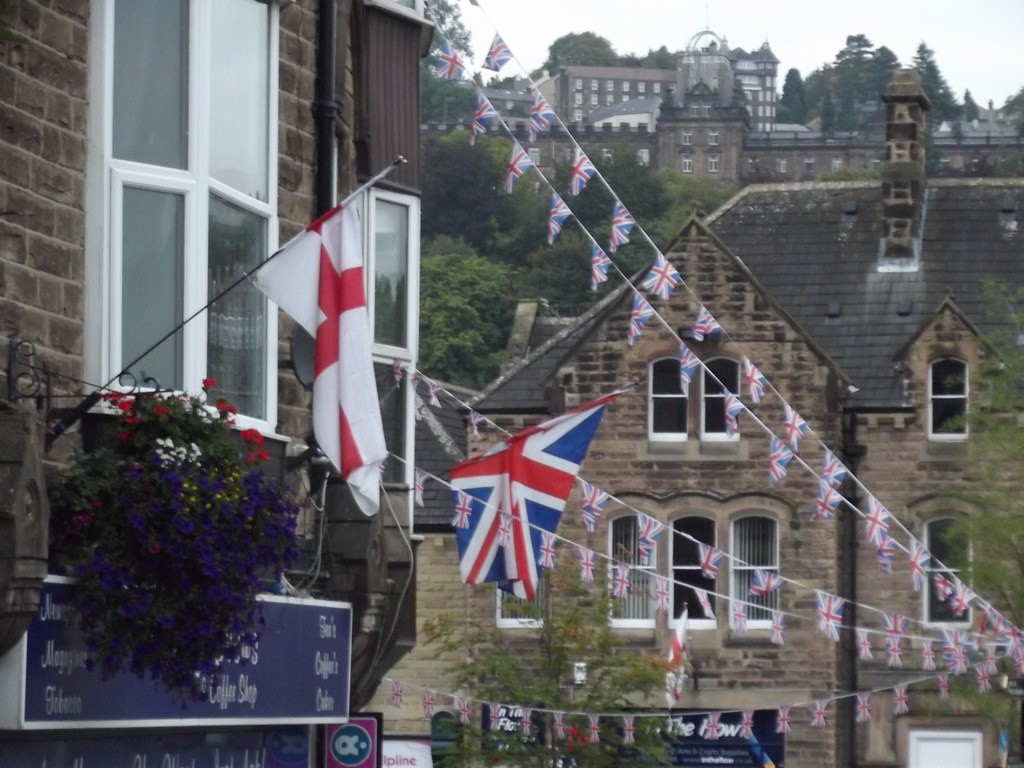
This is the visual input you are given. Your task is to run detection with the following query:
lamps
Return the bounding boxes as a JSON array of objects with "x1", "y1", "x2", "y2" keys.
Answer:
[{"x1": 677, "y1": 325, "x2": 708, "y2": 345}]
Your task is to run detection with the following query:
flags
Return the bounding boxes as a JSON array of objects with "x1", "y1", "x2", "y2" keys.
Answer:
[
  {"x1": 680, "y1": 343, "x2": 701, "y2": 399},
  {"x1": 666, "y1": 608, "x2": 688, "y2": 709},
  {"x1": 592, "y1": 242, "x2": 612, "y2": 292},
  {"x1": 502, "y1": 143, "x2": 533, "y2": 194},
  {"x1": 527, "y1": 91, "x2": 554, "y2": 140},
  {"x1": 743, "y1": 356, "x2": 766, "y2": 404},
  {"x1": 389, "y1": 677, "x2": 910, "y2": 744},
  {"x1": 261, "y1": 188, "x2": 387, "y2": 518},
  {"x1": 468, "y1": 92, "x2": 499, "y2": 146},
  {"x1": 412, "y1": 403, "x2": 1024, "y2": 702},
  {"x1": 724, "y1": 388, "x2": 745, "y2": 437},
  {"x1": 570, "y1": 149, "x2": 595, "y2": 196},
  {"x1": 548, "y1": 193, "x2": 573, "y2": 246},
  {"x1": 448, "y1": 390, "x2": 626, "y2": 602},
  {"x1": 643, "y1": 251, "x2": 679, "y2": 301},
  {"x1": 694, "y1": 307, "x2": 722, "y2": 341},
  {"x1": 435, "y1": 39, "x2": 463, "y2": 80},
  {"x1": 610, "y1": 201, "x2": 635, "y2": 254},
  {"x1": 627, "y1": 294, "x2": 654, "y2": 346},
  {"x1": 482, "y1": 32, "x2": 515, "y2": 72}
]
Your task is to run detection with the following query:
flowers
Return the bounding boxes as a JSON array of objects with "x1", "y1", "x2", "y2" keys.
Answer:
[{"x1": 42, "y1": 370, "x2": 305, "y2": 711}]
